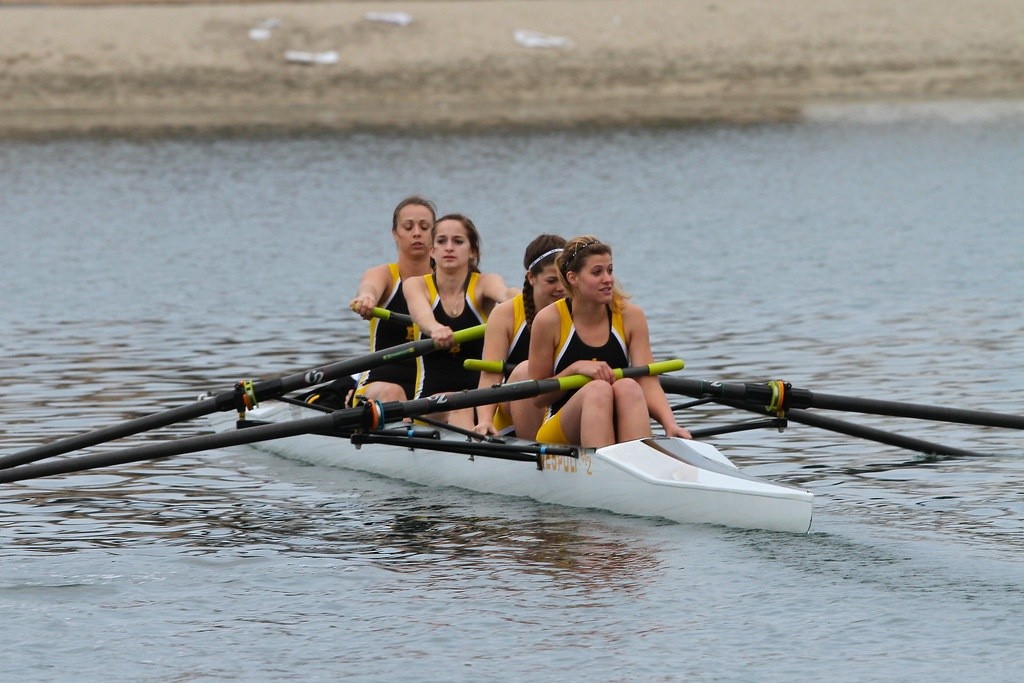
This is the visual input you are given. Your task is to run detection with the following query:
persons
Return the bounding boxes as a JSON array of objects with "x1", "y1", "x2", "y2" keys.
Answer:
[
  {"x1": 403, "y1": 214, "x2": 521, "y2": 432},
  {"x1": 348, "y1": 195, "x2": 436, "y2": 418},
  {"x1": 529, "y1": 236, "x2": 692, "y2": 451},
  {"x1": 469, "y1": 234, "x2": 568, "y2": 439}
]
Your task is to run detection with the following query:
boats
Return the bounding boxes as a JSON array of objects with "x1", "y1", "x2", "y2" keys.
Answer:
[{"x1": 194, "y1": 384, "x2": 815, "y2": 538}]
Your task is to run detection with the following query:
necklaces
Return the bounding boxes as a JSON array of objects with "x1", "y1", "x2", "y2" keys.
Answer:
[{"x1": 439, "y1": 289, "x2": 463, "y2": 316}]
